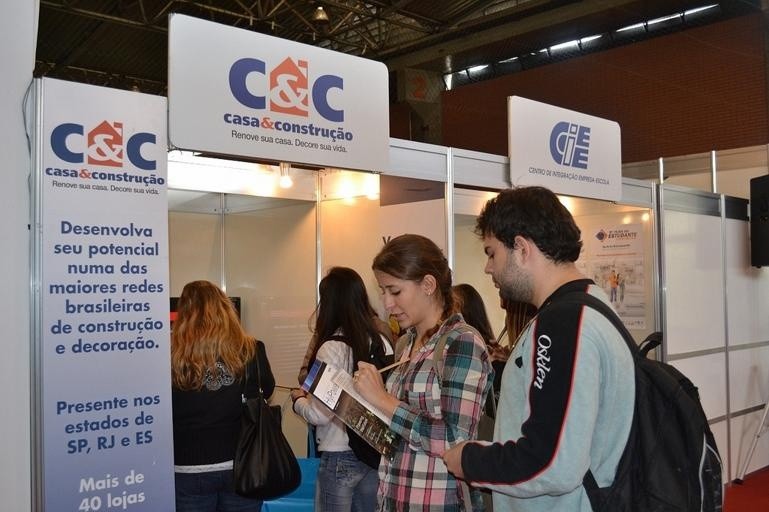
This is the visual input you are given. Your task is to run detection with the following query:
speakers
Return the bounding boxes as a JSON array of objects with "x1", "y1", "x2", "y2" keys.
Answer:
[{"x1": 750, "y1": 174, "x2": 769, "y2": 268}]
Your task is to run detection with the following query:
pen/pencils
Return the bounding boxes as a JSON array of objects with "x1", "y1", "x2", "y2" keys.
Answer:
[{"x1": 377, "y1": 358, "x2": 409, "y2": 374}]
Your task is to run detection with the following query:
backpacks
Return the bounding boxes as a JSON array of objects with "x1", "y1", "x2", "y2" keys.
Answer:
[
  {"x1": 547, "y1": 292, "x2": 723, "y2": 512},
  {"x1": 309, "y1": 335, "x2": 395, "y2": 471},
  {"x1": 395, "y1": 324, "x2": 495, "y2": 512}
]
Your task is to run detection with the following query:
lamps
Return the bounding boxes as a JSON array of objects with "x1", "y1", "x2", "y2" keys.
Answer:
[{"x1": 311, "y1": 2, "x2": 329, "y2": 24}]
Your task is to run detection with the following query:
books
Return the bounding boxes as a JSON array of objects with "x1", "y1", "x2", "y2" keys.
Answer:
[{"x1": 300, "y1": 358, "x2": 399, "y2": 463}]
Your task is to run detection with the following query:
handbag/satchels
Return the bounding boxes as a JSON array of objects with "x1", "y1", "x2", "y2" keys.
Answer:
[{"x1": 233, "y1": 338, "x2": 301, "y2": 500}]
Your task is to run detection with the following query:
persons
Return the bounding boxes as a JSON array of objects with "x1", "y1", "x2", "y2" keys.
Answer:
[
  {"x1": 502, "y1": 296, "x2": 538, "y2": 352},
  {"x1": 442, "y1": 185, "x2": 723, "y2": 512},
  {"x1": 450, "y1": 285, "x2": 508, "y2": 420},
  {"x1": 352, "y1": 233, "x2": 498, "y2": 512},
  {"x1": 170, "y1": 281, "x2": 301, "y2": 512},
  {"x1": 290, "y1": 267, "x2": 395, "y2": 512}
]
